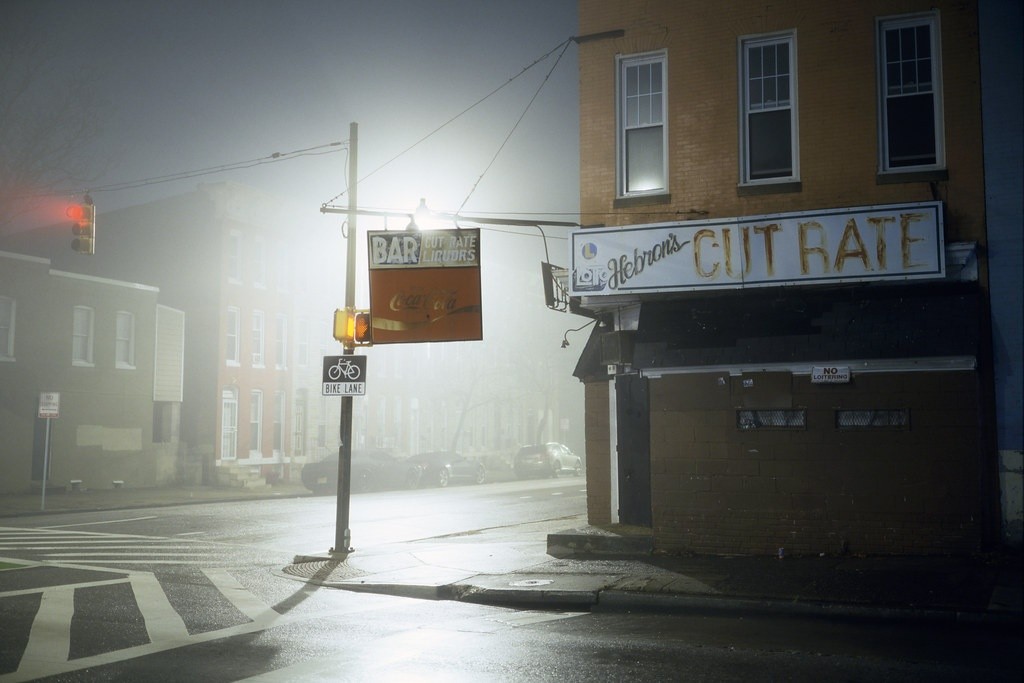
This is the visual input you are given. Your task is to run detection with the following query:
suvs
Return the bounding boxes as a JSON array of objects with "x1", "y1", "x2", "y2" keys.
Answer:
[{"x1": 513, "y1": 442, "x2": 584, "y2": 479}]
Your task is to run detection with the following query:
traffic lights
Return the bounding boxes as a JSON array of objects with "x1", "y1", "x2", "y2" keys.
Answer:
[
  {"x1": 355, "y1": 312, "x2": 371, "y2": 344},
  {"x1": 333, "y1": 309, "x2": 354, "y2": 342},
  {"x1": 65, "y1": 204, "x2": 96, "y2": 255}
]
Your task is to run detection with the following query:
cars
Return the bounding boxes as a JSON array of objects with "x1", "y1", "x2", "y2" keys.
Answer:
[
  {"x1": 301, "y1": 446, "x2": 419, "y2": 494},
  {"x1": 411, "y1": 448, "x2": 487, "y2": 488}
]
